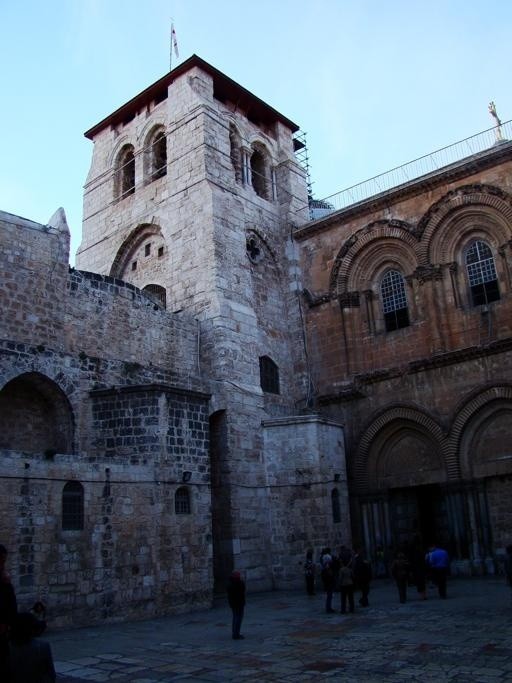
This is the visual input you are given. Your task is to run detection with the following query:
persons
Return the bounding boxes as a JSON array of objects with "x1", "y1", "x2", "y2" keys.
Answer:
[
  {"x1": 303, "y1": 544, "x2": 450, "y2": 614},
  {"x1": 0, "y1": 544, "x2": 46, "y2": 683},
  {"x1": 227, "y1": 570, "x2": 247, "y2": 640}
]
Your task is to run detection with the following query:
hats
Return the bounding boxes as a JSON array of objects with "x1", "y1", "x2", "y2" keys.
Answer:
[
  {"x1": 5, "y1": 613, "x2": 48, "y2": 638},
  {"x1": 231, "y1": 570, "x2": 241, "y2": 578}
]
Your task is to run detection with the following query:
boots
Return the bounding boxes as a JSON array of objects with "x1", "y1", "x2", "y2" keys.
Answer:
[{"x1": 326, "y1": 597, "x2": 335, "y2": 613}]
